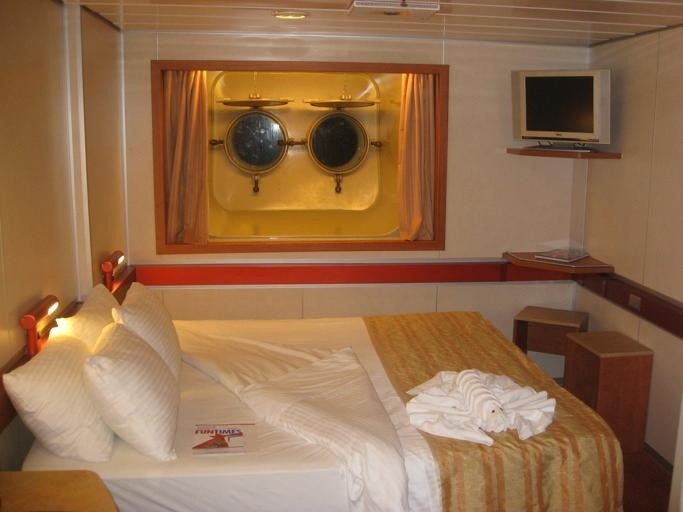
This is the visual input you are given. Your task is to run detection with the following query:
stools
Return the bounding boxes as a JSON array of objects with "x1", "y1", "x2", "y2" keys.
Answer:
[{"x1": 514, "y1": 304, "x2": 591, "y2": 357}]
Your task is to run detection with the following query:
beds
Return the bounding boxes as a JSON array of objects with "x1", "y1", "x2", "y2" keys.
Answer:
[{"x1": 0, "y1": 297, "x2": 626, "y2": 512}]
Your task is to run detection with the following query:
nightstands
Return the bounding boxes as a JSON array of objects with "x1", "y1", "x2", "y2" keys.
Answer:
[
  {"x1": 565, "y1": 329, "x2": 654, "y2": 455},
  {"x1": 1, "y1": 471, "x2": 119, "y2": 512}
]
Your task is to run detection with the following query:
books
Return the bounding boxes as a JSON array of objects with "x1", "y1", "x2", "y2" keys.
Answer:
[
  {"x1": 532, "y1": 249, "x2": 592, "y2": 262},
  {"x1": 191, "y1": 426, "x2": 246, "y2": 451}
]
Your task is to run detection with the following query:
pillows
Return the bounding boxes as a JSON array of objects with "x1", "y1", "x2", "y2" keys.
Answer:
[
  {"x1": 2, "y1": 326, "x2": 115, "y2": 464},
  {"x1": 82, "y1": 323, "x2": 181, "y2": 465},
  {"x1": 55, "y1": 283, "x2": 120, "y2": 351},
  {"x1": 111, "y1": 281, "x2": 180, "y2": 382}
]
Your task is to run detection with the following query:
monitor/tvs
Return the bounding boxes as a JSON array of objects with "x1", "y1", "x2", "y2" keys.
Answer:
[{"x1": 520, "y1": 69, "x2": 610, "y2": 152}]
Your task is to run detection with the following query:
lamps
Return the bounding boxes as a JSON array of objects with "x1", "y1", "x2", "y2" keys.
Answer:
[
  {"x1": 19, "y1": 294, "x2": 61, "y2": 360},
  {"x1": 102, "y1": 249, "x2": 126, "y2": 291}
]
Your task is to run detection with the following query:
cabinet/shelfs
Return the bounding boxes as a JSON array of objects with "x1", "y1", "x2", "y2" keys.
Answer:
[
  {"x1": 506, "y1": 141, "x2": 623, "y2": 162},
  {"x1": 504, "y1": 251, "x2": 615, "y2": 275}
]
